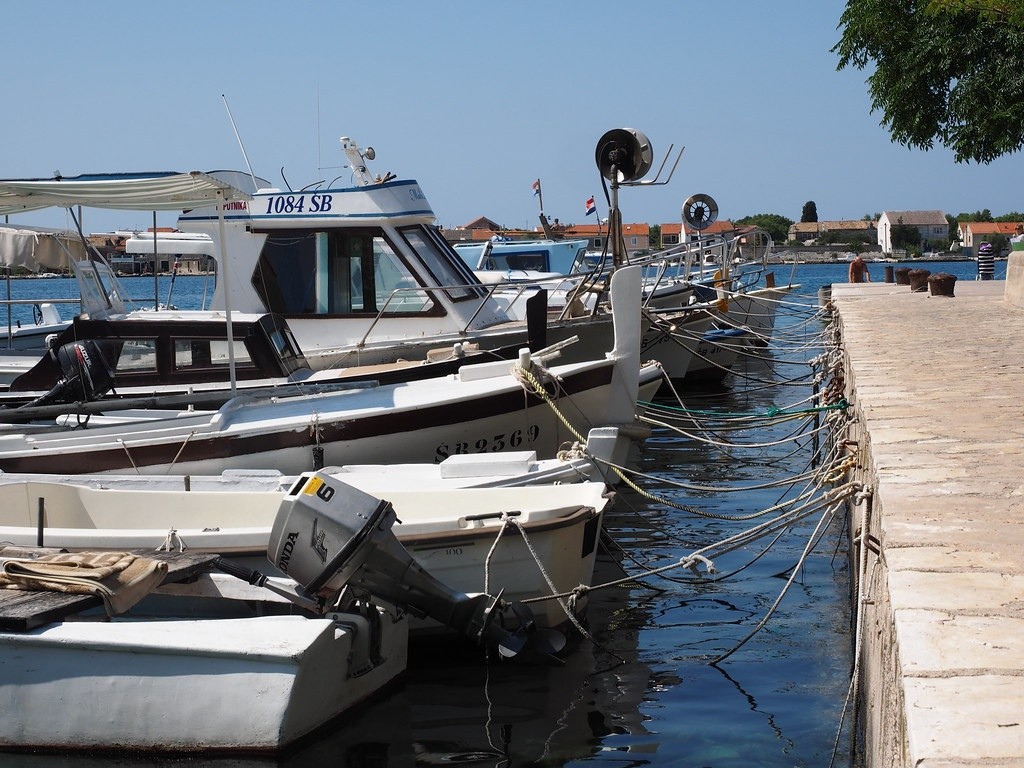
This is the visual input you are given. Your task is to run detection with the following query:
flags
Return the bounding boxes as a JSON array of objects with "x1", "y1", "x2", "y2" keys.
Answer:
[
  {"x1": 585, "y1": 196, "x2": 596, "y2": 216},
  {"x1": 531, "y1": 180, "x2": 539, "y2": 196}
]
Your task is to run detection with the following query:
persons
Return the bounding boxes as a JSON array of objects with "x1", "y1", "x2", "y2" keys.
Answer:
[{"x1": 848, "y1": 256, "x2": 872, "y2": 283}]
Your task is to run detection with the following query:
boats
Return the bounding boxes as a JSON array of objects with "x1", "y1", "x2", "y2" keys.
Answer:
[{"x1": 0, "y1": 94, "x2": 806, "y2": 754}]
